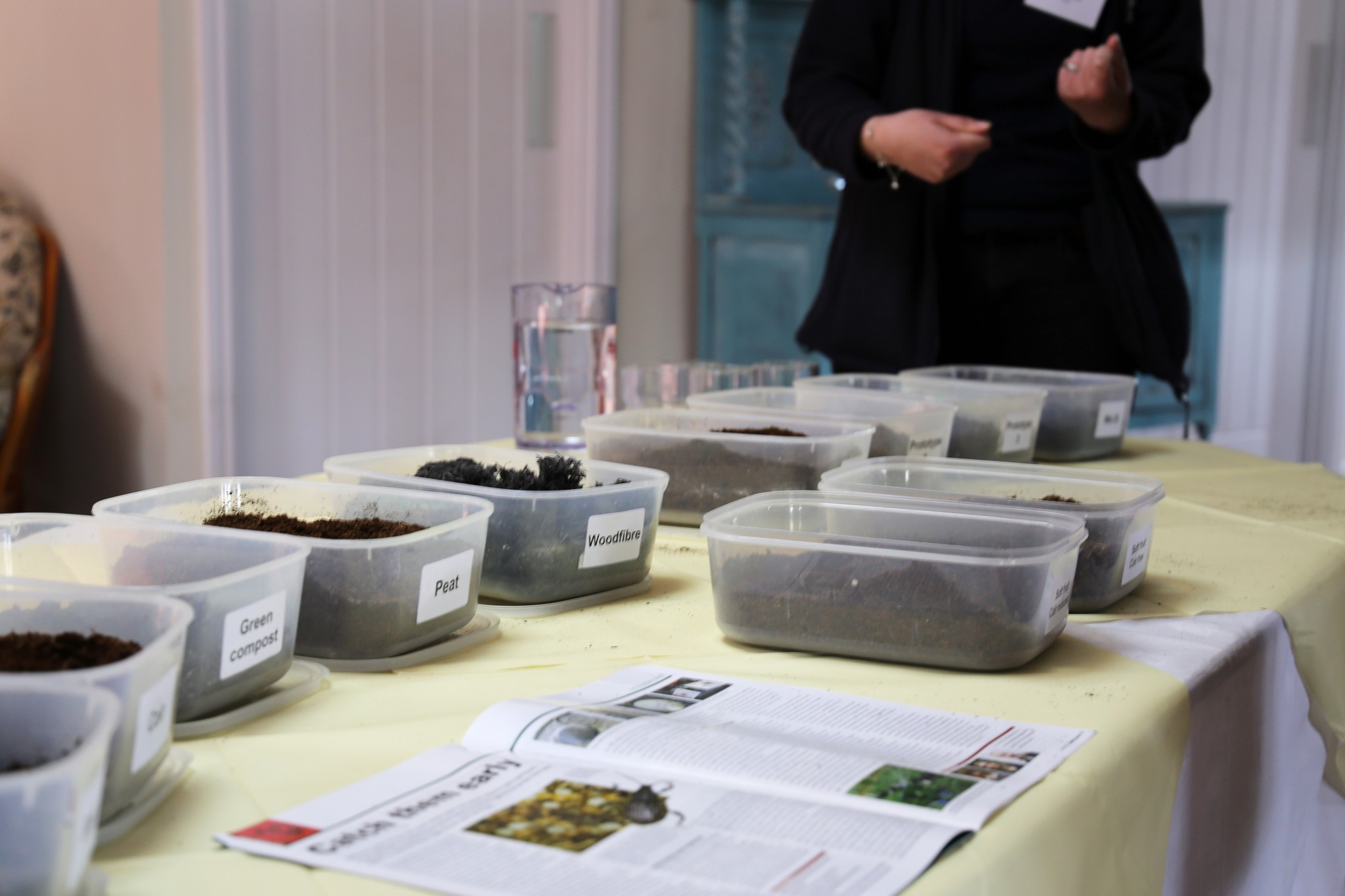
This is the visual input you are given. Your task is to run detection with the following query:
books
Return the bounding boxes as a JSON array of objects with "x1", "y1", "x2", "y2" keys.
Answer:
[{"x1": 210, "y1": 664, "x2": 1098, "y2": 896}]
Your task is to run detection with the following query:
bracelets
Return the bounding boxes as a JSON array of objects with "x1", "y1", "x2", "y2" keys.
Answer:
[{"x1": 864, "y1": 115, "x2": 898, "y2": 189}]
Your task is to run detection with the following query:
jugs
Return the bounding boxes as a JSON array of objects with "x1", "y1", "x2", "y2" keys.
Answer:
[{"x1": 511, "y1": 280, "x2": 619, "y2": 451}]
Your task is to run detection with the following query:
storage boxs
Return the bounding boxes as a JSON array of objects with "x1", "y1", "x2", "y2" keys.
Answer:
[{"x1": 0, "y1": 365, "x2": 1165, "y2": 896}]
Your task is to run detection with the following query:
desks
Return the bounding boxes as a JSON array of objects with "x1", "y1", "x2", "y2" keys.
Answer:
[{"x1": 0, "y1": 438, "x2": 1345, "y2": 896}]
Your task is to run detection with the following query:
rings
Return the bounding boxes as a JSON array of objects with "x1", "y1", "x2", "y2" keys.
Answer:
[{"x1": 1064, "y1": 62, "x2": 1079, "y2": 71}]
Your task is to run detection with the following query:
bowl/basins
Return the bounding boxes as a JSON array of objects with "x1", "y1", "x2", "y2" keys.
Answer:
[
  {"x1": 0, "y1": 584, "x2": 195, "y2": 826},
  {"x1": 898, "y1": 364, "x2": 1140, "y2": 460},
  {"x1": 91, "y1": 475, "x2": 494, "y2": 660},
  {"x1": 793, "y1": 373, "x2": 1048, "y2": 463},
  {"x1": 0, "y1": 679, "x2": 120, "y2": 896},
  {"x1": 325, "y1": 443, "x2": 669, "y2": 605},
  {"x1": 686, "y1": 386, "x2": 958, "y2": 458},
  {"x1": 0, "y1": 513, "x2": 311, "y2": 722},
  {"x1": 818, "y1": 455, "x2": 1169, "y2": 613},
  {"x1": 580, "y1": 408, "x2": 877, "y2": 527},
  {"x1": 699, "y1": 491, "x2": 1090, "y2": 668}
]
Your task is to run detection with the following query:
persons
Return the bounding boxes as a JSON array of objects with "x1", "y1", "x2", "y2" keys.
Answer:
[{"x1": 779, "y1": 0, "x2": 1212, "y2": 442}]
